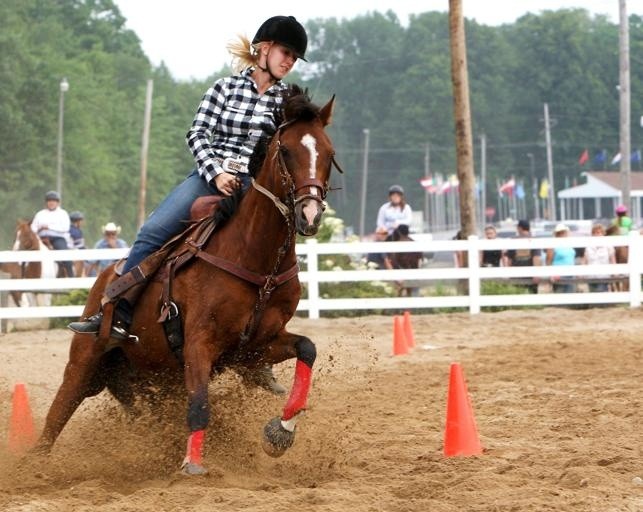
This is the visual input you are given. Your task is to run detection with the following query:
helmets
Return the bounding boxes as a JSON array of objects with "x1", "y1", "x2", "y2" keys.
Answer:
[
  {"x1": 44, "y1": 190, "x2": 62, "y2": 202},
  {"x1": 250, "y1": 14, "x2": 310, "y2": 65},
  {"x1": 387, "y1": 183, "x2": 404, "y2": 197},
  {"x1": 69, "y1": 210, "x2": 84, "y2": 221}
]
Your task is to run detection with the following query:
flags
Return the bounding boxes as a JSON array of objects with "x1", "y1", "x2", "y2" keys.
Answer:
[
  {"x1": 418, "y1": 172, "x2": 550, "y2": 200},
  {"x1": 595, "y1": 150, "x2": 606, "y2": 163},
  {"x1": 578, "y1": 149, "x2": 588, "y2": 165},
  {"x1": 611, "y1": 152, "x2": 621, "y2": 166},
  {"x1": 631, "y1": 149, "x2": 641, "y2": 161}
]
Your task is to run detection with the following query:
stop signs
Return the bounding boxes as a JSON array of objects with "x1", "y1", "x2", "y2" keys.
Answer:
[{"x1": 486, "y1": 206, "x2": 495, "y2": 217}]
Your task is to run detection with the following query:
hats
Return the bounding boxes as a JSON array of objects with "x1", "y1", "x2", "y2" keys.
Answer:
[
  {"x1": 99, "y1": 221, "x2": 122, "y2": 237},
  {"x1": 613, "y1": 204, "x2": 628, "y2": 213},
  {"x1": 516, "y1": 218, "x2": 530, "y2": 229},
  {"x1": 552, "y1": 223, "x2": 571, "y2": 235}
]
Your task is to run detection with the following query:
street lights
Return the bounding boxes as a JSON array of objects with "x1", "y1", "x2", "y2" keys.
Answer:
[{"x1": 57, "y1": 76, "x2": 71, "y2": 207}]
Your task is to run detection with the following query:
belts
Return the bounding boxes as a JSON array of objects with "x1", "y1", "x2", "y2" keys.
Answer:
[{"x1": 213, "y1": 154, "x2": 252, "y2": 177}]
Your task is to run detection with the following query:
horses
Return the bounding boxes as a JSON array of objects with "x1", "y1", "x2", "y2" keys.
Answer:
[
  {"x1": 12, "y1": 82, "x2": 336, "y2": 476},
  {"x1": 11, "y1": 217, "x2": 87, "y2": 330}
]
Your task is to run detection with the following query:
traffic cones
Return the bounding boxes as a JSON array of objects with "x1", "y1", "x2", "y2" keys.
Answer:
[
  {"x1": 402, "y1": 311, "x2": 416, "y2": 349},
  {"x1": 7, "y1": 381, "x2": 36, "y2": 453},
  {"x1": 393, "y1": 316, "x2": 409, "y2": 355},
  {"x1": 442, "y1": 359, "x2": 483, "y2": 458}
]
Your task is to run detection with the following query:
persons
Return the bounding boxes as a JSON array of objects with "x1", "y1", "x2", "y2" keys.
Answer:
[
  {"x1": 82, "y1": 222, "x2": 129, "y2": 277},
  {"x1": 69, "y1": 16, "x2": 308, "y2": 394},
  {"x1": 452, "y1": 206, "x2": 633, "y2": 293},
  {"x1": 70, "y1": 211, "x2": 84, "y2": 249},
  {"x1": 376, "y1": 185, "x2": 413, "y2": 271},
  {"x1": 31, "y1": 192, "x2": 74, "y2": 277}
]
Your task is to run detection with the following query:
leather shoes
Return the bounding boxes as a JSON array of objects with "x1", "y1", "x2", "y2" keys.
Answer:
[{"x1": 65, "y1": 306, "x2": 132, "y2": 342}]
